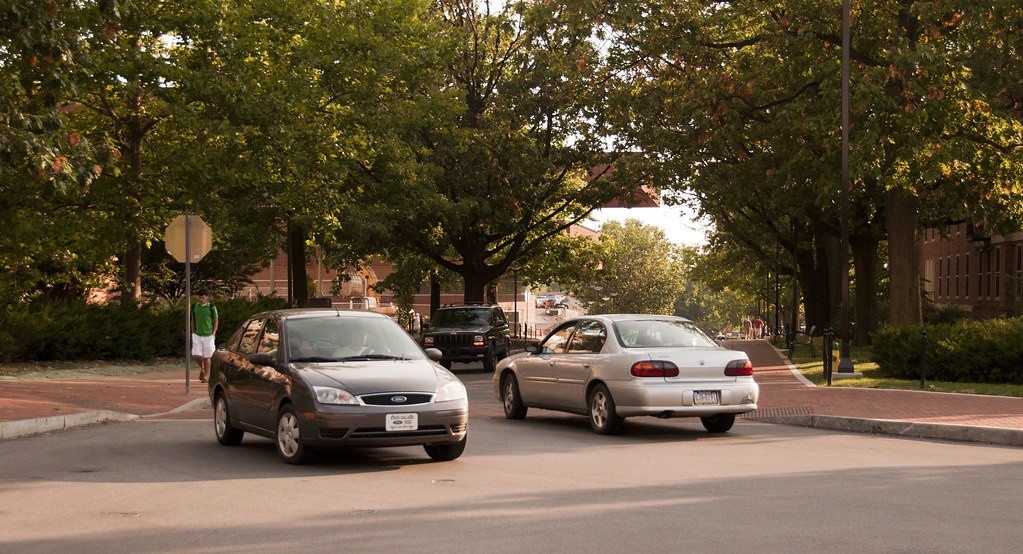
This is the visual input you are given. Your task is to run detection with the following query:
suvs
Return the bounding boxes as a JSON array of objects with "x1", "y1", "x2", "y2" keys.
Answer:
[{"x1": 423, "y1": 301, "x2": 512, "y2": 372}]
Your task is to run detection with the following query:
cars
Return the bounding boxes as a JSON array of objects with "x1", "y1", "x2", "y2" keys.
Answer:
[
  {"x1": 493, "y1": 315, "x2": 761, "y2": 435},
  {"x1": 205, "y1": 308, "x2": 470, "y2": 467}
]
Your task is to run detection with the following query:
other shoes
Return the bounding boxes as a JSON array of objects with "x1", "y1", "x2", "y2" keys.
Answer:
[
  {"x1": 199, "y1": 370, "x2": 204, "y2": 380},
  {"x1": 202, "y1": 377, "x2": 210, "y2": 383}
]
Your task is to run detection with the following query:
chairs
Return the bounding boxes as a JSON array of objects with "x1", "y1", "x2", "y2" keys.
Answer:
[{"x1": 788, "y1": 325, "x2": 817, "y2": 360}]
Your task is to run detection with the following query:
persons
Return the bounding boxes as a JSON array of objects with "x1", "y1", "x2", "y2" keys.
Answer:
[
  {"x1": 190, "y1": 289, "x2": 218, "y2": 383},
  {"x1": 330, "y1": 326, "x2": 375, "y2": 359},
  {"x1": 753, "y1": 316, "x2": 764, "y2": 342},
  {"x1": 744, "y1": 315, "x2": 753, "y2": 341}
]
